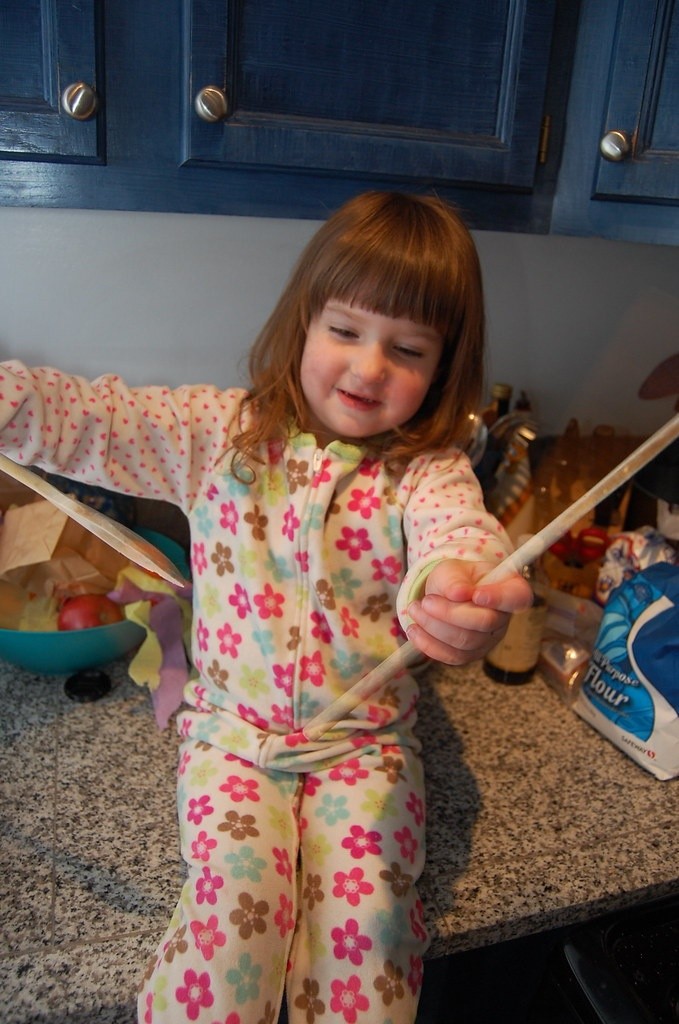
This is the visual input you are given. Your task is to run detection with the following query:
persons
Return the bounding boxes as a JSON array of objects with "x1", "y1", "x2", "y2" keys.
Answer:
[{"x1": 0, "y1": 191, "x2": 534, "y2": 1024}]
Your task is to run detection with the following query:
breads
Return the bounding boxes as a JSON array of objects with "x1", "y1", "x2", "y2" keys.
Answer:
[{"x1": 536, "y1": 635, "x2": 590, "y2": 698}]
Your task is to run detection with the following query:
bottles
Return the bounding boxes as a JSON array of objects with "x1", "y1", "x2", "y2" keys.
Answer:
[
  {"x1": 482, "y1": 533, "x2": 547, "y2": 685},
  {"x1": 473, "y1": 383, "x2": 513, "y2": 499}
]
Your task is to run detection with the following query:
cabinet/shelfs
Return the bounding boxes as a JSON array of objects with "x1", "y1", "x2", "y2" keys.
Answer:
[{"x1": 0, "y1": 1, "x2": 679, "y2": 248}]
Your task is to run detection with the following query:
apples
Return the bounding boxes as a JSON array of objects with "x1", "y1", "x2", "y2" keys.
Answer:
[{"x1": 56, "y1": 593, "x2": 123, "y2": 631}]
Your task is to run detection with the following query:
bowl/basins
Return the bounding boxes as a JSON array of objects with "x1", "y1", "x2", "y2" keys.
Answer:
[{"x1": 0, "y1": 525, "x2": 189, "y2": 675}]
